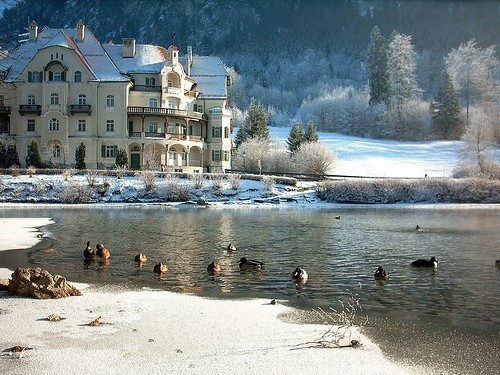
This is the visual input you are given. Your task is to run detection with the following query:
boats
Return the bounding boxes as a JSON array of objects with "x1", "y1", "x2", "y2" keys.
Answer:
[{"x1": 197, "y1": 197, "x2": 208, "y2": 205}]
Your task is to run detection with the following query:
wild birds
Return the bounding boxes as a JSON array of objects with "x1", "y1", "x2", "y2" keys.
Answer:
[
  {"x1": 335, "y1": 216, "x2": 341, "y2": 219},
  {"x1": 83, "y1": 240, "x2": 95, "y2": 261},
  {"x1": 135, "y1": 253, "x2": 147, "y2": 263},
  {"x1": 95, "y1": 243, "x2": 111, "y2": 261},
  {"x1": 153, "y1": 261, "x2": 168, "y2": 274},
  {"x1": 415, "y1": 225, "x2": 422, "y2": 231},
  {"x1": 410, "y1": 257, "x2": 438, "y2": 269},
  {"x1": 237, "y1": 256, "x2": 263, "y2": 271},
  {"x1": 292, "y1": 267, "x2": 309, "y2": 285},
  {"x1": 374, "y1": 265, "x2": 389, "y2": 278},
  {"x1": 227, "y1": 244, "x2": 237, "y2": 252},
  {"x1": 207, "y1": 261, "x2": 221, "y2": 272}
]
